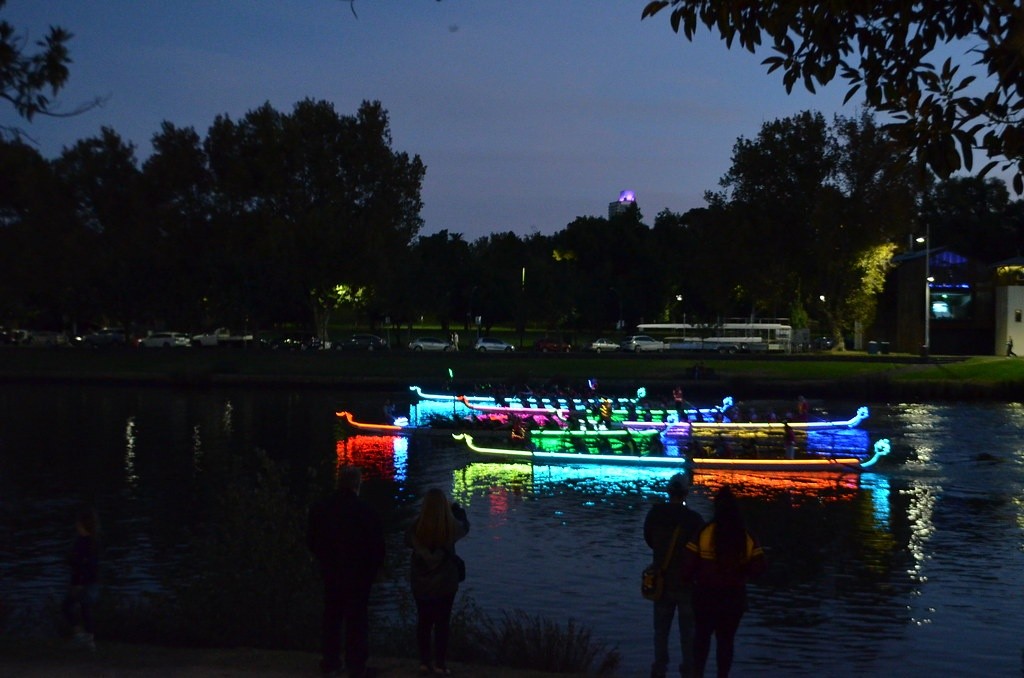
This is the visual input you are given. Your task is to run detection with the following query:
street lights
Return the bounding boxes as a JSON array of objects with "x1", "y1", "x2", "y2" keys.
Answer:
[{"x1": 915, "y1": 235, "x2": 935, "y2": 347}]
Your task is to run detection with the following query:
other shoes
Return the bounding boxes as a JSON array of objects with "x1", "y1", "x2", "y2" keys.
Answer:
[
  {"x1": 325, "y1": 659, "x2": 342, "y2": 673},
  {"x1": 62, "y1": 633, "x2": 96, "y2": 652},
  {"x1": 352, "y1": 667, "x2": 378, "y2": 677}
]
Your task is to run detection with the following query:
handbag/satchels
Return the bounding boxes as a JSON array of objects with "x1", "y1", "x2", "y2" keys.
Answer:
[
  {"x1": 445, "y1": 556, "x2": 466, "y2": 584},
  {"x1": 641, "y1": 563, "x2": 670, "y2": 600}
]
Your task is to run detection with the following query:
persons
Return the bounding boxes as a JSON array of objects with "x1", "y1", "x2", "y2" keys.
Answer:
[
  {"x1": 683, "y1": 486, "x2": 767, "y2": 678},
  {"x1": 448, "y1": 331, "x2": 460, "y2": 352},
  {"x1": 33, "y1": 505, "x2": 99, "y2": 650},
  {"x1": 303, "y1": 465, "x2": 386, "y2": 678},
  {"x1": 643, "y1": 476, "x2": 706, "y2": 678},
  {"x1": 403, "y1": 486, "x2": 470, "y2": 676}
]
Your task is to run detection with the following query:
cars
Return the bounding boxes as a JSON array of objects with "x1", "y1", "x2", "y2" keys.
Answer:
[
  {"x1": 71, "y1": 326, "x2": 132, "y2": 347},
  {"x1": 331, "y1": 334, "x2": 388, "y2": 351},
  {"x1": 534, "y1": 338, "x2": 573, "y2": 353},
  {"x1": 475, "y1": 336, "x2": 515, "y2": 352},
  {"x1": 802, "y1": 335, "x2": 833, "y2": 352},
  {"x1": 137, "y1": 332, "x2": 187, "y2": 349},
  {"x1": 3, "y1": 323, "x2": 72, "y2": 347},
  {"x1": 580, "y1": 338, "x2": 620, "y2": 353},
  {"x1": 620, "y1": 336, "x2": 664, "y2": 353},
  {"x1": 271, "y1": 331, "x2": 324, "y2": 352},
  {"x1": 185, "y1": 326, "x2": 254, "y2": 348},
  {"x1": 408, "y1": 336, "x2": 456, "y2": 352}
]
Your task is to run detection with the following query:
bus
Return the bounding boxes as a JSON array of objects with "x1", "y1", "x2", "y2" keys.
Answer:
[{"x1": 636, "y1": 323, "x2": 792, "y2": 354}]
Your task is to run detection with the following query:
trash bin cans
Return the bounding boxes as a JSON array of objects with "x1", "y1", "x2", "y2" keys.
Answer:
[
  {"x1": 881, "y1": 342, "x2": 891, "y2": 354},
  {"x1": 868, "y1": 342, "x2": 877, "y2": 354}
]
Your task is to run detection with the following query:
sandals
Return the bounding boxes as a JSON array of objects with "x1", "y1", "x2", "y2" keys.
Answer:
[
  {"x1": 418, "y1": 663, "x2": 433, "y2": 673},
  {"x1": 434, "y1": 665, "x2": 452, "y2": 675}
]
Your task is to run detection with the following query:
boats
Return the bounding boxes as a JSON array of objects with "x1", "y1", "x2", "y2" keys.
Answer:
[
  {"x1": 450, "y1": 395, "x2": 892, "y2": 474},
  {"x1": 336, "y1": 385, "x2": 733, "y2": 440}
]
